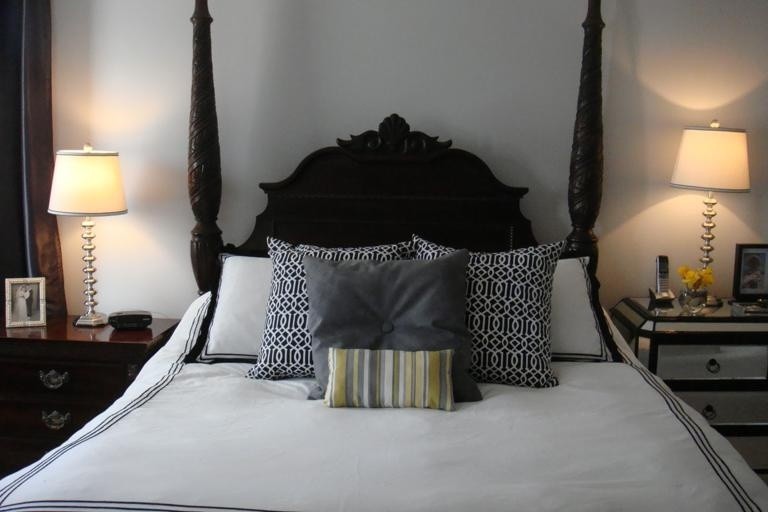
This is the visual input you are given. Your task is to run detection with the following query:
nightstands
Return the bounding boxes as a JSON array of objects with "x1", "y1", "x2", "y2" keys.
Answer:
[
  {"x1": 607, "y1": 295, "x2": 768, "y2": 486},
  {"x1": 0, "y1": 313, "x2": 181, "y2": 480}
]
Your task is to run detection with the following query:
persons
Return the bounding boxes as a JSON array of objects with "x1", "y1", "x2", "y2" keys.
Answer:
[
  {"x1": 12, "y1": 284, "x2": 28, "y2": 321},
  {"x1": 24, "y1": 283, "x2": 34, "y2": 318},
  {"x1": 743, "y1": 254, "x2": 762, "y2": 288}
]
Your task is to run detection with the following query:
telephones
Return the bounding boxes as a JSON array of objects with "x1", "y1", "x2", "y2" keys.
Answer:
[{"x1": 649, "y1": 255, "x2": 676, "y2": 305}]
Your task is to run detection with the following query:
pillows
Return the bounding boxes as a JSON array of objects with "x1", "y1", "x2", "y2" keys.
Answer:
[
  {"x1": 324, "y1": 344, "x2": 454, "y2": 411},
  {"x1": 189, "y1": 239, "x2": 271, "y2": 364},
  {"x1": 246, "y1": 235, "x2": 414, "y2": 380},
  {"x1": 411, "y1": 234, "x2": 567, "y2": 392},
  {"x1": 545, "y1": 244, "x2": 619, "y2": 364},
  {"x1": 300, "y1": 251, "x2": 481, "y2": 403}
]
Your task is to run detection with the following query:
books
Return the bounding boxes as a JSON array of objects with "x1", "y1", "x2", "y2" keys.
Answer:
[{"x1": 733, "y1": 301, "x2": 768, "y2": 314}]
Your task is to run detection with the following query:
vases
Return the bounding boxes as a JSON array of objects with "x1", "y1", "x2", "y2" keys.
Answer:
[{"x1": 682, "y1": 289, "x2": 708, "y2": 307}]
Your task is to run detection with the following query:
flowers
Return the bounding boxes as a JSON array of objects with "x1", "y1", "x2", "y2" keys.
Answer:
[{"x1": 675, "y1": 264, "x2": 715, "y2": 292}]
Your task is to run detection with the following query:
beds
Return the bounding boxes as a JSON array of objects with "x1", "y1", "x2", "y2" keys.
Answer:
[{"x1": 0, "y1": 0, "x2": 767, "y2": 512}]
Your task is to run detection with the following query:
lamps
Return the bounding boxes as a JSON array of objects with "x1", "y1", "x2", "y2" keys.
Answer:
[
  {"x1": 668, "y1": 118, "x2": 753, "y2": 308},
  {"x1": 46, "y1": 142, "x2": 129, "y2": 328}
]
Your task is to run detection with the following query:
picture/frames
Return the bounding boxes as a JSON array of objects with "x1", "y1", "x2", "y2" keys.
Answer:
[
  {"x1": 5, "y1": 276, "x2": 47, "y2": 329},
  {"x1": 732, "y1": 242, "x2": 768, "y2": 301}
]
